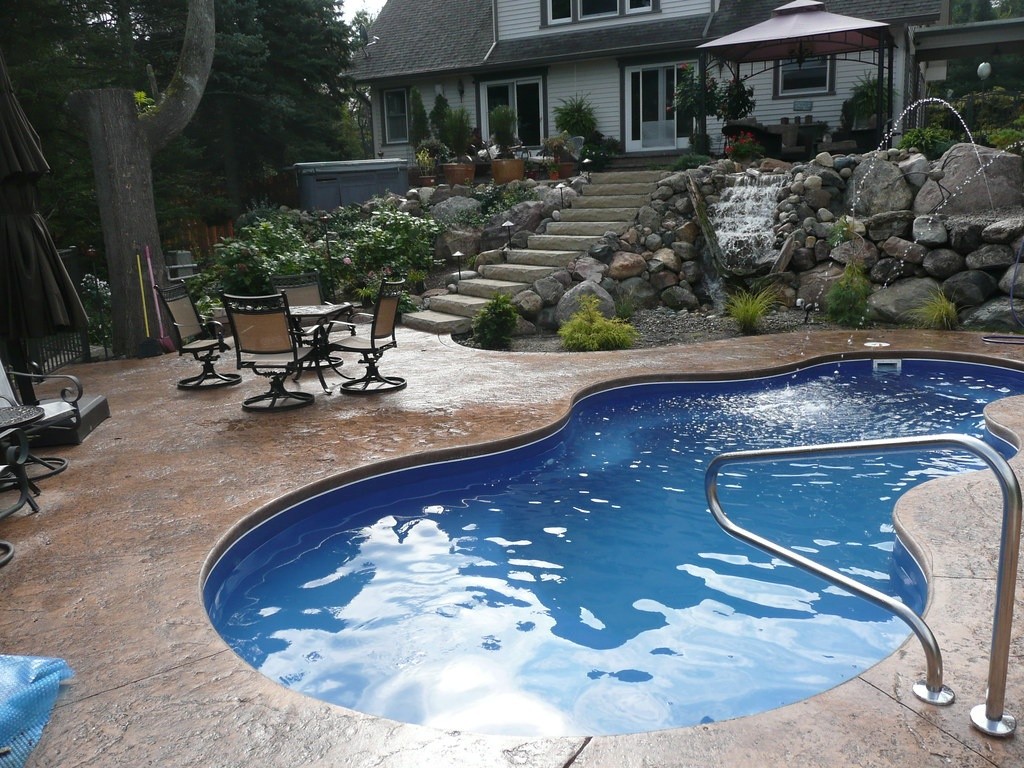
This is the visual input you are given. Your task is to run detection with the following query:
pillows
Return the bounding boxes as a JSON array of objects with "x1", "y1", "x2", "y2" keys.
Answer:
[
  {"x1": 767, "y1": 123, "x2": 799, "y2": 147},
  {"x1": 728, "y1": 117, "x2": 756, "y2": 127}
]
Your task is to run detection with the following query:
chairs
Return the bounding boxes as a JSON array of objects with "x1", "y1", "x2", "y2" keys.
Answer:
[
  {"x1": 0, "y1": 360, "x2": 84, "y2": 482},
  {"x1": 153, "y1": 278, "x2": 243, "y2": 391},
  {"x1": 327, "y1": 278, "x2": 407, "y2": 395},
  {"x1": 0, "y1": 428, "x2": 40, "y2": 518},
  {"x1": 565, "y1": 136, "x2": 584, "y2": 176},
  {"x1": 267, "y1": 271, "x2": 343, "y2": 371},
  {"x1": 831, "y1": 127, "x2": 882, "y2": 154},
  {"x1": 218, "y1": 288, "x2": 315, "y2": 413}
]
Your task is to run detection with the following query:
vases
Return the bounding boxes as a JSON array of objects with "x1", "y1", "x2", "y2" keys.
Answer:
[
  {"x1": 418, "y1": 176, "x2": 436, "y2": 187},
  {"x1": 556, "y1": 162, "x2": 575, "y2": 180}
]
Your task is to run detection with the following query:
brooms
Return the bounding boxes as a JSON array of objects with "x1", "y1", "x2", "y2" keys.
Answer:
[{"x1": 131, "y1": 244, "x2": 176, "y2": 359}]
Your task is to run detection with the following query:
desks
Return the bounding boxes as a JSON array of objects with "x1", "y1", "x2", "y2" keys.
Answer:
[
  {"x1": 765, "y1": 122, "x2": 824, "y2": 137},
  {"x1": 0, "y1": 406, "x2": 56, "y2": 496},
  {"x1": 238, "y1": 304, "x2": 356, "y2": 394}
]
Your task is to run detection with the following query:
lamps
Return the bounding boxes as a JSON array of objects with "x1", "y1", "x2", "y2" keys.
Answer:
[
  {"x1": 582, "y1": 158, "x2": 592, "y2": 176},
  {"x1": 501, "y1": 220, "x2": 515, "y2": 250},
  {"x1": 452, "y1": 250, "x2": 466, "y2": 280},
  {"x1": 556, "y1": 183, "x2": 566, "y2": 209}
]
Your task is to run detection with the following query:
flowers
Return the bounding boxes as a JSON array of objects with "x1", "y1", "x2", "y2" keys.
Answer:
[
  {"x1": 415, "y1": 146, "x2": 436, "y2": 176},
  {"x1": 542, "y1": 130, "x2": 576, "y2": 162},
  {"x1": 723, "y1": 131, "x2": 764, "y2": 163}
]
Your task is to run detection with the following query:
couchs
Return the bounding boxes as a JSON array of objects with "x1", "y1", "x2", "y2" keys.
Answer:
[{"x1": 722, "y1": 125, "x2": 861, "y2": 162}]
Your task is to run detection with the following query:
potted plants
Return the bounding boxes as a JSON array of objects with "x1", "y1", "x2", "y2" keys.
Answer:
[
  {"x1": 524, "y1": 159, "x2": 537, "y2": 179},
  {"x1": 849, "y1": 68, "x2": 902, "y2": 128},
  {"x1": 489, "y1": 104, "x2": 525, "y2": 185},
  {"x1": 438, "y1": 104, "x2": 476, "y2": 187},
  {"x1": 548, "y1": 161, "x2": 559, "y2": 180}
]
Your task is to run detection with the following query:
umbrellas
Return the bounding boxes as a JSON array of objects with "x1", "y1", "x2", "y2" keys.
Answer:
[{"x1": 0, "y1": 58, "x2": 89, "y2": 406}]
